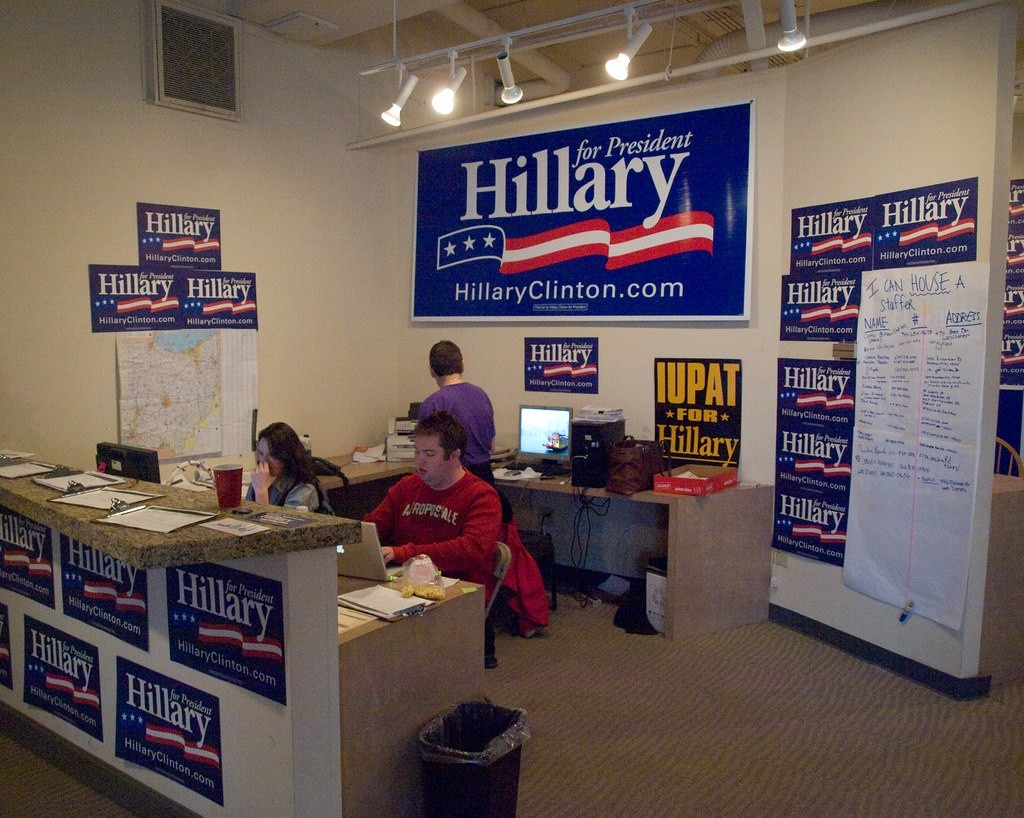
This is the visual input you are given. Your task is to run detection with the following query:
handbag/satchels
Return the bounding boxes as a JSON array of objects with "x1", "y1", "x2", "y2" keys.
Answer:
[{"x1": 604, "y1": 435, "x2": 672, "y2": 496}]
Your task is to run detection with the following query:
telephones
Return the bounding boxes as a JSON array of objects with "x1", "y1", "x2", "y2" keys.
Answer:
[{"x1": 312, "y1": 457, "x2": 340, "y2": 478}]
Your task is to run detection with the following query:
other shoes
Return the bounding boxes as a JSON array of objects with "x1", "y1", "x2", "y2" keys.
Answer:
[{"x1": 485, "y1": 656, "x2": 498, "y2": 668}]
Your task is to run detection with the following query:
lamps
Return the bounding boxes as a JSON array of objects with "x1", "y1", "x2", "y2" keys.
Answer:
[
  {"x1": 432, "y1": 52, "x2": 467, "y2": 114},
  {"x1": 778, "y1": 0, "x2": 806, "y2": 51},
  {"x1": 381, "y1": 64, "x2": 419, "y2": 126},
  {"x1": 605, "y1": 6, "x2": 652, "y2": 80},
  {"x1": 496, "y1": 37, "x2": 523, "y2": 105}
]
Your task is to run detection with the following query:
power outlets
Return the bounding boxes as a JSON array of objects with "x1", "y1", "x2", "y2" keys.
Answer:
[
  {"x1": 537, "y1": 505, "x2": 554, "y2": 526},
  {"x1": 567, "y1": 496, "x2": 588, "y2": 508}
]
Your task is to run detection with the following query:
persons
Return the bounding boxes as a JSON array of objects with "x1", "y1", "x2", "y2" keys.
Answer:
[
  {"x1": 361, "y1": 415, "x2": 503, "y2": 616},
  {"x1": 246, "y1": 422, "x2": 320, "y2": 514},
  {"x1": 415, "y1": 340, "x2": 501, "y2": 670}
]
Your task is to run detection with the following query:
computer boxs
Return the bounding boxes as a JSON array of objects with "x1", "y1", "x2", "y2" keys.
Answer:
[{"x1": 571, "y1": 419, "x2": 627, "y2": 488}]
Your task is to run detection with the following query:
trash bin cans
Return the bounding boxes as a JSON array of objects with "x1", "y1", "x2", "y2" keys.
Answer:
[
  {"x1": 643, "y1": 555, "x2": 669, "y2": 635},
  {"x1": 417, "y1": 700, "x2": 526, "y2": 818}
]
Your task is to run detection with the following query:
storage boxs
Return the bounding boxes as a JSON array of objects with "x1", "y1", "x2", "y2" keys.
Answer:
[{"x1": 653, "y1": 465, "x2": 739, "y2": 495}]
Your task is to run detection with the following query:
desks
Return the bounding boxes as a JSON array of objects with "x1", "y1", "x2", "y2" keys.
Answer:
[{"x1": 194, "y1": 452, "x2": 772, "y2": 818}]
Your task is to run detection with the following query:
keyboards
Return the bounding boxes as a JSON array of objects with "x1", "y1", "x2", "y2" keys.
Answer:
[{"x1": 503, "y1": 462, "x2": 572, "y2": 477}]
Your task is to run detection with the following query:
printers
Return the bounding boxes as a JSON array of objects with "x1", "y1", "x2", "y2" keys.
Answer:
[{"x1": 387, "y1": 402, "x2": 422, "y2": 462}]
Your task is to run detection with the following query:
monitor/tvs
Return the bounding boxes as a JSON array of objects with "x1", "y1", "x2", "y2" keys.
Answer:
[
  {"x1": 95, "y1": 442, "x2": 161, "y2": 485},
  {"x1": 517, "y1": 404, "x2": 573, "y2": 468}
]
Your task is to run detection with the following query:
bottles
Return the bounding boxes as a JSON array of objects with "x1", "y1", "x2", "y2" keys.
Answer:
[{"x1": 302, "y1": 435, "x2": 312, "y2": 457}]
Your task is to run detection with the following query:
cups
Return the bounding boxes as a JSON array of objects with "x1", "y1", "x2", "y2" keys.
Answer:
[{"x1": 213, "y1": 463, "x2": 242, "y2": 508}]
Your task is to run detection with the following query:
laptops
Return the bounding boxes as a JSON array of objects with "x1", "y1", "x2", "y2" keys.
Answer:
[{"x1": 335, "y1": 522, "x2": 404, "y2": 582}]
